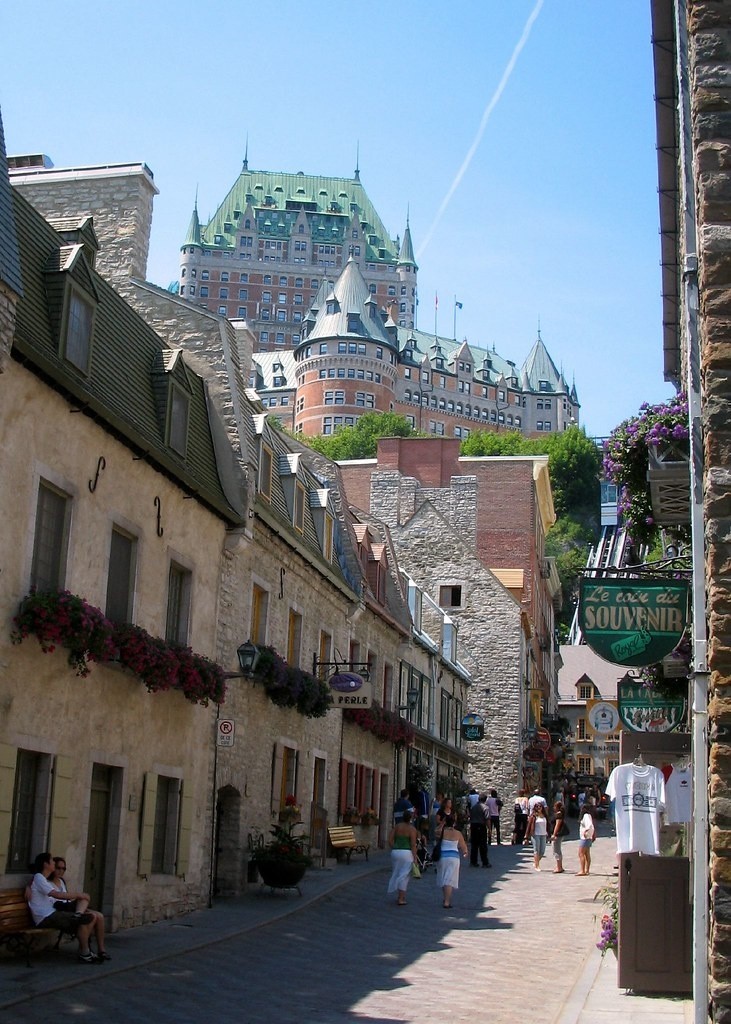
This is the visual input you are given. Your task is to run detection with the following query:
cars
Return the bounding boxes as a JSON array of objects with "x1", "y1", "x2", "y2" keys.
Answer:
[{"x1": 568, "y1": 770, "x2": 608, "y2": 817}]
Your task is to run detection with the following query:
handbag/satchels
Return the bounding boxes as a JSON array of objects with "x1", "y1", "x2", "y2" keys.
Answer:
[
  {"x1": 434, "y1": 821, "x2": 446, "y2": 835},
  {"x1": 412, "y1": 862, "x2": 422, "y2": 879},
  {"x1": 432, "y1": 828, "x2": 444, "y2": 862},
  {"x1": 555, "y1": 816, "x2": 570, "y2": 836}
]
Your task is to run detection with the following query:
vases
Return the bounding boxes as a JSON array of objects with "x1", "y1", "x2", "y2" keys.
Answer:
[
  {"x1": 610, "y1": 945, "x2": 619, "y2": 959},
  {"x1": 342, "y1": 814, "x2": 362, "y2": 824},
  {"x1": 256, "y1": 860, "x2": 307, "y2": 898},
  {"x1": 362, "y1": 817, "x2": 376, "y2": 825},
  {"x1": 279, "y1": 811, "x2": 302, "y2": 823}
]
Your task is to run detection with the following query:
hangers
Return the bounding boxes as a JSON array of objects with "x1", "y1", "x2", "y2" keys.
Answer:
[{"x1": 634, "y1": 744, "x2": 647, "y2": 767}]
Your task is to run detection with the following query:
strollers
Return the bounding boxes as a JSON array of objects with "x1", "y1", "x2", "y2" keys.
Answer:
[{"x1": 416, "y1": 837, "x2": 438, "y2": 874}]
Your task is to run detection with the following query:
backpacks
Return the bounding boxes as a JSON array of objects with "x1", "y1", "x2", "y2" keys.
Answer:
[
  {"x1": 514, "y1": 797, "x2": 524, "y2": 813},
  {"x1": 471, "y1": 802, "x2": 487, "y2": 822}
]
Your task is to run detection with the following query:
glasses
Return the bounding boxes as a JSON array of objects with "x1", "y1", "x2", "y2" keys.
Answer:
[{"x1": 55, "y1": 866, "x2": 66, "y2": 870}]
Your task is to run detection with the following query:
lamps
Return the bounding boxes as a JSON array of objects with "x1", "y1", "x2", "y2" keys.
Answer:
[
  {"x1": 394, "y1": 687, "x2": 419, "y2": 710},
  {"x1": 223, "y1": 640, "x2": 261, "y2": 681},
  {"x1": 313, "y1": 652, "x2": 373, "y2": 683}
]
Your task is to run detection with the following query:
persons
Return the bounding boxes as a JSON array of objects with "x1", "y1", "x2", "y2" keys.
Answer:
[
  {"x1": 574, "y1": 804, "x2": 595, "y2": 876},
  {"x1": 392, "y1": 788, "x2": 416, "y2": 825},
  {"x1": 551, "y1": 768, "x2": 608, "y2": 816},
  {"x1": 512, "y1": 789, "x2": 549, "y2": 845},
  {"x1": 524, "y1": 801, "x2": 549, "y2": 872},
  {"x1": 417, "y1": 831, "x2": 432, "y2": 861},
  {"x1": 24, "y1": 853, "x2": 112, "y2": 962},
  {"x1": 469, "y1": 789, "x2": 479, "y2": 809},
  {"x1": 387, "y1": 810, "x2": 418, "y2": 904},
  {"x1": 428, "y1": 790, "x2": 470, "y2": 834},
  {"x1": 434, "y1": 814, "x2": 468, "y2": 907},
  {"x1": 487, "y1": 789, "x2": 505, "y2": 845},
  {"x1": 469, "y1": 794, "x2": 492, "y2": 868},
  {"x1": 550, "y1": 801, "x2": 565, "y2": 873}
]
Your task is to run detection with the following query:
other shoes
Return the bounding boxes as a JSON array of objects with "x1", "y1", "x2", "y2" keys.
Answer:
[
  {"x1": 575, "y1": 872, "x2": 589, "y2": 875},
  {"x1": 553, "y1": 867, "x2": 563, "y2": 873},
  {"x1": 482, "y1": 862, "x2": 491, "y2": 867},
  {"x1": 443, "y1": 899, "x2": 453, "y2": 908},
  {"x1": 398, "y1": 897, "x2": 409, "y2": 904},
  {"x1": 532, "y1": 863, "x2": 542, "y2": 872},
  {"x1": 98, "y1": 952, "x2": 112, "y2": 959},
  {"x1": 470, "y1": 863, "x2": 480, "y2": 866}
]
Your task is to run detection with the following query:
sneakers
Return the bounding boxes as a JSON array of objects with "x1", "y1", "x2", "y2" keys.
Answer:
[
  {"x1": 71, "y1": 913, "x2": 94, "y2": 924},
  {"x1": 80, "y1": 951, "x2": 104, "y2": 964}
]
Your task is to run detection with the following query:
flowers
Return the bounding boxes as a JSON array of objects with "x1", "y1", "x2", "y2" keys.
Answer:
[
  {"x1": 285, "y1": 793, "x2": 302, "y2": 813},
  {"x1": 251, "y1": 823, "x2": 313, "y2": 868},
  {"x1": 343, "y1": 699, "x2": 414, "y2": 754},
  {"x1": 436, "y1": 774, "x2": 471, "y2": 797},
  {"x1": 11, "y1": 588, "x2": 227, "y2": 708},
  {"x1": 256, "y1": 642, "x2": 334, "y2": 720},
  {"x1": 408, "y1": 761, "x2": 435, "y2": 792},
  {"x1": 600, "y1": 390, "x2": 691, "y2": 694},
  {"x1": 346, "y1": 802, "x2": 382, "y2": 825},
  {"x1": 596, "y1": 914, "x2": 619, "y2": 958}
]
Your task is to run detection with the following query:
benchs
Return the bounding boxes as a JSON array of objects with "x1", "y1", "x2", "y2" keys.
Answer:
[
  {"x1": 328, "y1": 825, "x2": 371, "y2": 865},
  {"x1": 0, "y1": 888, "x2": 94, "y2": 968}
]
